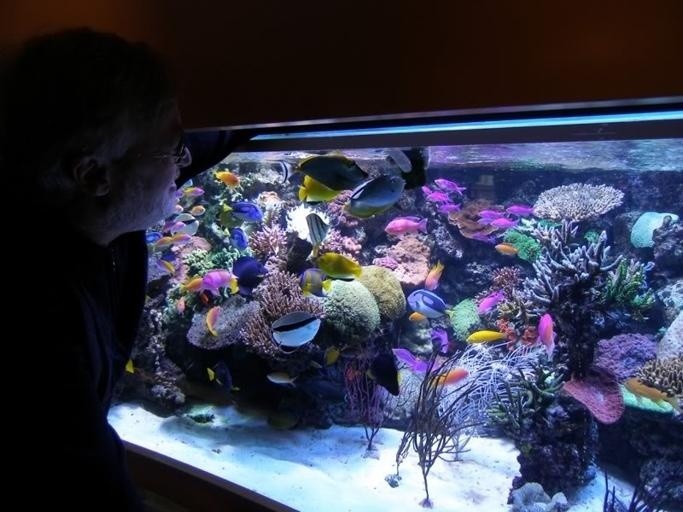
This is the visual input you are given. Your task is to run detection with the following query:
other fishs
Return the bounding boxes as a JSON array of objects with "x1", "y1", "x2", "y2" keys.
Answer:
[{"x1": 142, "y1": 148, "x2": 561, "y2": 390}]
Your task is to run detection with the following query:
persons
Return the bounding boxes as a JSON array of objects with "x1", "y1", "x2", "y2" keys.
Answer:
[{"x1": 2, "y1": 28, "x2": 196, "y2": 509}]
